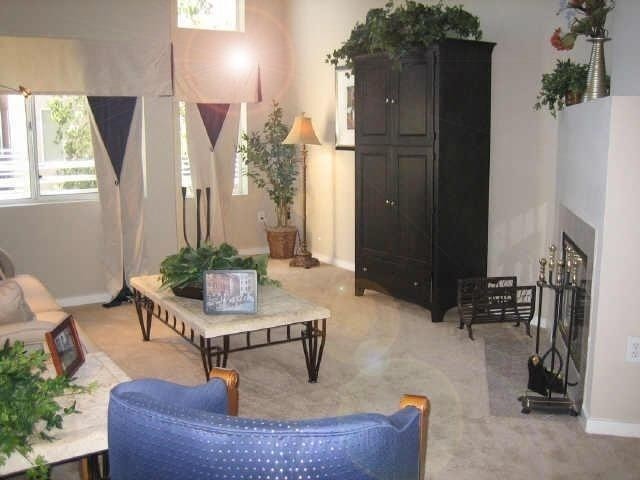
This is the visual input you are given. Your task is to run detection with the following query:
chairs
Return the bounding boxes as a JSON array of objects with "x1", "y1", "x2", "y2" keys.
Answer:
[{"x1": 107, "y1": 366, "x2": 432, "y2": 480}]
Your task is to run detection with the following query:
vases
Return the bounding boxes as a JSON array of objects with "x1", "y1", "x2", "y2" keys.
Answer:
[{"x1": 582, "y1": 37, "x2": 614, "y2": 101}]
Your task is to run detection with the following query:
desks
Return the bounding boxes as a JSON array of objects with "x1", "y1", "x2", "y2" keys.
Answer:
[{"x1": 0, "y1": 352, "x2": 133, "y2": 479}]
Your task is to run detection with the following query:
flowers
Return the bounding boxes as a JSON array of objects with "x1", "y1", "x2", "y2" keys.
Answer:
[{"x1": 548, "y1": 0, "x2": 615, "y2": 50}]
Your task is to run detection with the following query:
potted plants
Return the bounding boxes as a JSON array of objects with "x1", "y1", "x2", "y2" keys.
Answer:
[
  {"x1": 234, "y1": 99, "x2": 301, "y2": 260},
  {"x1": 155, "y1": 241, "x2": 282, "y2": 299},
  {"x1": 535, "y1": 57, "x2": 611, "y2": 117}
]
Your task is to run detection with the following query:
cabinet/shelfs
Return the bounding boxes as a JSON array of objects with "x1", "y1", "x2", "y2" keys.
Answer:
[{"x1": 348, "y1": 35, "x2": 498, "y2": 325}]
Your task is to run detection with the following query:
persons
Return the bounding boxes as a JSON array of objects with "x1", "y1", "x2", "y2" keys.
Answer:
[{"x1": 56, "y1": 333, "x2": 74, "y2": 355}]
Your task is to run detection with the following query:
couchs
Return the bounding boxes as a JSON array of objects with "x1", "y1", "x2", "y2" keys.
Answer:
[{"x1": 0, "y1": 249, "x2": 102, "y2": 372}]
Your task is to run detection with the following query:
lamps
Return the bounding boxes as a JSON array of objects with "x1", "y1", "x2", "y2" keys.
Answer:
[{"x1": 278, "y1": 111, "x2": 324, "y2": 268}]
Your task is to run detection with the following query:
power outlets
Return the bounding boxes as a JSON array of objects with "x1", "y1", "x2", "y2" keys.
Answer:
[{"x1": 257, "y1": 210, "x2": 265, "y2": 224}]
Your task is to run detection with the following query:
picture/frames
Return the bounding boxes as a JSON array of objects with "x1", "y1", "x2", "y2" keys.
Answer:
[
  {"x1": 201, "y1": 269, "x2": 259, "y2": 316},
  {"x1": 44, "y1": 314, "x2": 86, "y2": 381},
  {"x1": 333, "y1": 64, "x2": 354, "y2": 152}
]
